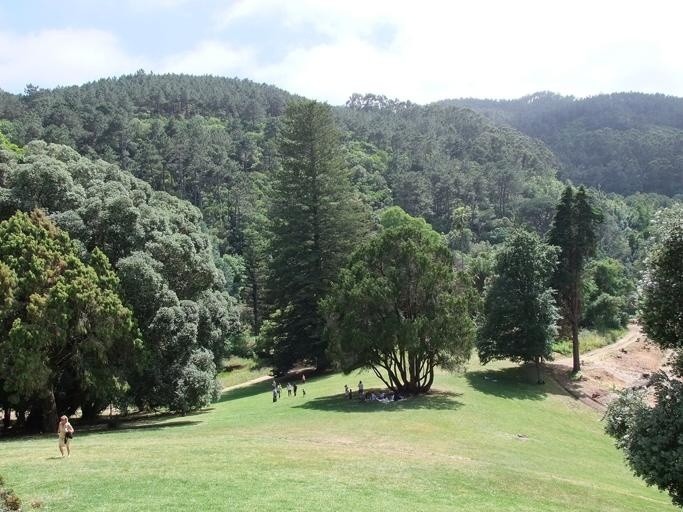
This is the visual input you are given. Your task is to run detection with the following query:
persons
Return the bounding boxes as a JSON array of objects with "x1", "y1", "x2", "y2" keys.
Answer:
[
  {"x1": 293, "y1": 384, "x2": 297, "y2": 396},
  {"x1": 272, "y1": 381, "x2": 282, "y2": 402},
  {"x1": 303, "y1": 389, "x2": 305, "y2": 398},
  {"x1": 345, "y1": 381, "x2": 398, "y2": 402},
  {"x1": 287, "y1": 383, "x2": 291, "y2": 396},
  {"x1": 302, "y1": 374, "x2": 305, "y2": 384},
  {"x1": 57, "y1": 415, "x2": 74, "y2": 458}
]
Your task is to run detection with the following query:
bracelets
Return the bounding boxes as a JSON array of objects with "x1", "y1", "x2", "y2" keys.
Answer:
[{"x1": 58, "y1": 432, "x2": 60, "y2": 433}]
